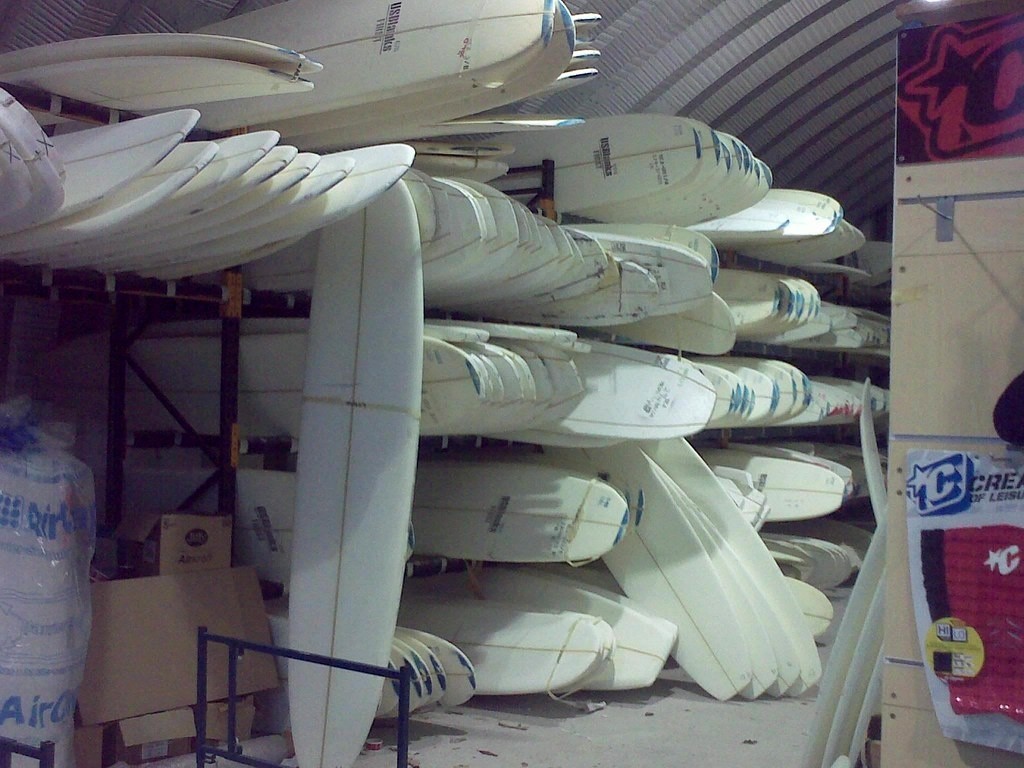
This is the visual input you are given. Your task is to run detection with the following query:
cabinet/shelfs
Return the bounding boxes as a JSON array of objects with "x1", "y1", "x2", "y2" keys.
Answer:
[{"x1": 0, "y1": 80, "x2": 884, "y2": 575}]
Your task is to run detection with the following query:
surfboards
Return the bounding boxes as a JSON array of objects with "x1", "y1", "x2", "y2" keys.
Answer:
[{"x1": 1, "y1": 0, "x2": 889, "y2": 762}]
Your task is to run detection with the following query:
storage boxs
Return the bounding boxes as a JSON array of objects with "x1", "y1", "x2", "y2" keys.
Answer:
[
  {"x1": 111, "y1": 507, "x2": 235, "y2": 578},
  {"x1": 191, "y1": 694, "x2": 255, "y2": 752},
  {"x1": 73, "y1": 732, "x2": 105, "y2": 766},
  {"x1": 75, "y1": 561, "x2": 278, "y2": 728},
  {"x1": 105, "y1": 707, "x2": 197, "y2": 766}
]
